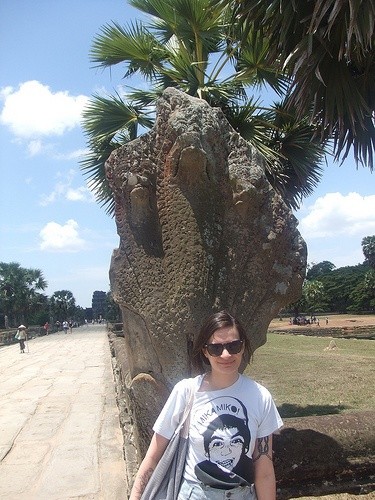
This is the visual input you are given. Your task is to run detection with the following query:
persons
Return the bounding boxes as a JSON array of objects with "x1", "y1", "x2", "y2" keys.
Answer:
[
  {"x1": 14, "y1": 316, "x2": 105, "y2": 354},
  {"x1": 129, "y1": 311, "x2": 285, "y2": 500},
  {"x1": 289, "y1": 313, "x2": 330, "y2": 327}
]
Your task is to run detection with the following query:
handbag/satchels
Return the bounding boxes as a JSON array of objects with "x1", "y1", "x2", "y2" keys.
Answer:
[{"x1": 141, "y1": 378, "x2": 194, "y2": 500}]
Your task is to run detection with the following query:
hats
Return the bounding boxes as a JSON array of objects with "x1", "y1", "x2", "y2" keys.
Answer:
[{"x1": 18, "y1": 325, "x2": 26, "y2": 328}]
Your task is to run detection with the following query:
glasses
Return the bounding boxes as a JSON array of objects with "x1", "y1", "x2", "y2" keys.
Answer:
[{"x1": 205, "y1": 339, "x2": 244, "y2": 357}]
